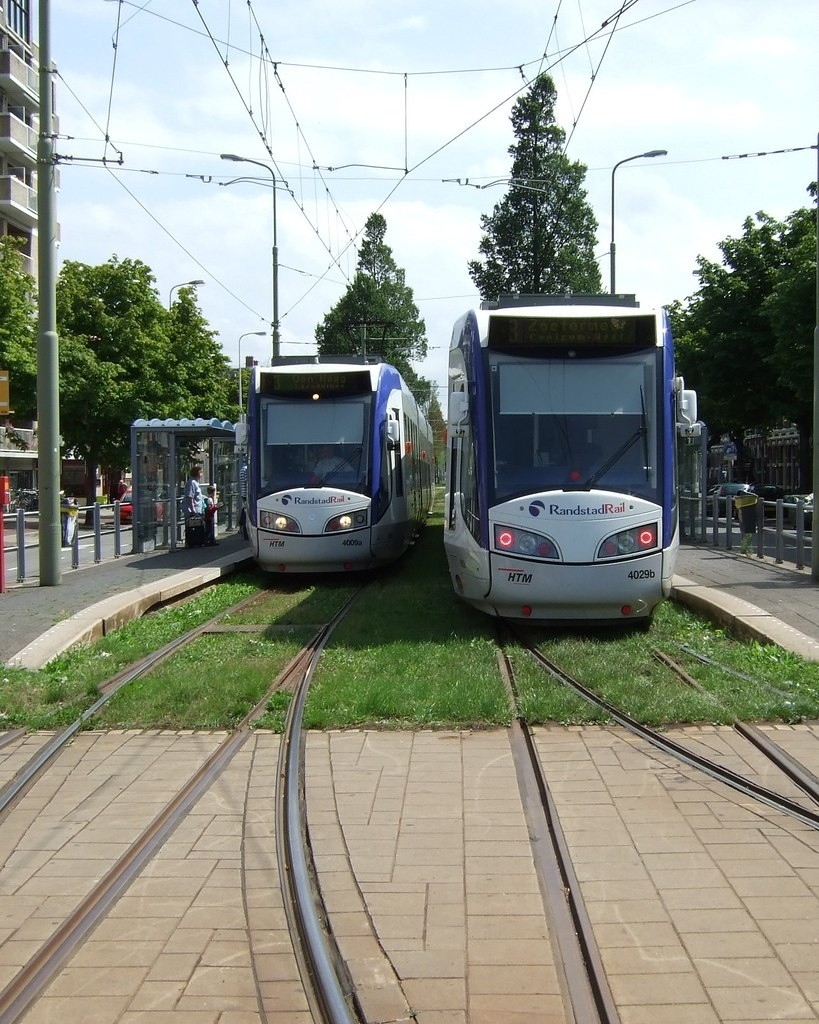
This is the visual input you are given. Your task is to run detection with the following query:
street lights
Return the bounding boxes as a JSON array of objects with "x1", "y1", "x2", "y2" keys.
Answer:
[
  {"x1": 610, "y1": 149, "x2": 668, "y2": 295},
  {"x1": 169, "y1": 279, "x2": 206, "y2": 311},
  {"x1": 220, "y1": 154, "x2": 279, "y2": 366},
  {"x1": 238, "y1": 332, "x2": 266, "y2": 408}
]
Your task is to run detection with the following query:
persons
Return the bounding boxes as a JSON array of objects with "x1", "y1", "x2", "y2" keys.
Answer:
[
  {"x1": 314, "y1": 445, "x2": 355, "y2": 480},
  {"x1": 183, "y1": 467, "x2": 207, "y2": 548},
  {"x1": 239, "y1": 465, "x2": 249, "y2": 540},
  {"x1": 204, "y1": 486, "x2": 220, "y2": 545},
  {"x1": 117, "y1": 480, "x2": 128, "y2": 499}
]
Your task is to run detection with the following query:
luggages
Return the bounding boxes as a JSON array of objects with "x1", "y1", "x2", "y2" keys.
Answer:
[{"x1": 184, "y1": 515, "x2": 207, "y2": 546}]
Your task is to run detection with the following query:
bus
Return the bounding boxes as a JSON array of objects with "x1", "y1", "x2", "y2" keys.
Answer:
[
  {"x1": 236, "y1": 354, "x2": 436, "y2": 573},
  {"x1": 444, "y1": 294, "x2": 703, "y2": 621}
]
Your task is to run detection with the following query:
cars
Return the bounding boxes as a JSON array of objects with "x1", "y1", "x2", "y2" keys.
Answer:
[
  {"x1": 707, "y1": 482, "x2": 814, "y2": 531},
  {"x1": 119, "y1": 486, "x2": 163, "y2": 524}
]
